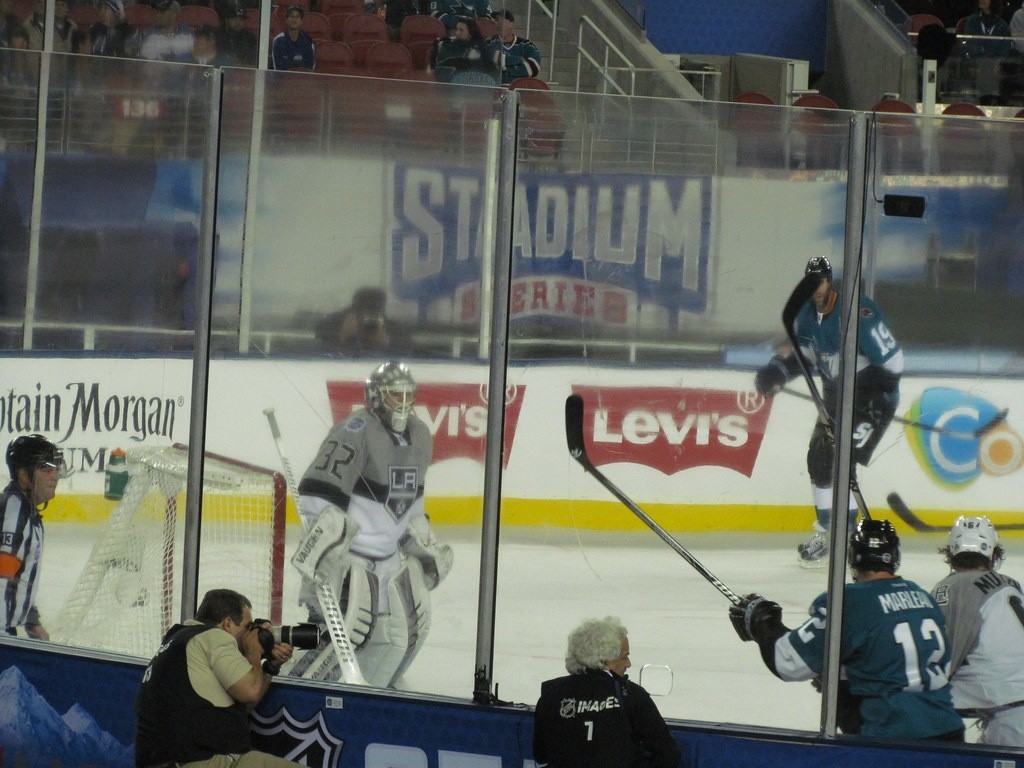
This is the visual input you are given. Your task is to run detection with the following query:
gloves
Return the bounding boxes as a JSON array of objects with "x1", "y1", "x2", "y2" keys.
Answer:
[
  {"x1": 755, "y1": 356, "x2": 792, "y2": 400},
  {"x1": 729, "y1": 592, "x2": 782, "y2": 641},
  {"x1": 860, "y1": 382, "x2": 899, "y2": 424}
]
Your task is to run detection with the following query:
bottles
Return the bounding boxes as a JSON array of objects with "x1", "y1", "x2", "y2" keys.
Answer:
[{"x1": 104, "y1": 447, "x2": 129, "y2": 501}]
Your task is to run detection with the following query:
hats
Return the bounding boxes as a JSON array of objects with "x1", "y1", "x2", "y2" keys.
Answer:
[
  {"x1": 151, "y1": 0, "x2": 181, "y2": 15},
  {"x1": 491, "y1": 9, "x2": 514, "y2": 23},
  {"x1": 224, "y1": 5, "x2": 249, "y2": 21}
]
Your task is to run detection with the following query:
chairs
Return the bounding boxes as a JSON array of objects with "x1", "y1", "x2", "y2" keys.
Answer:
[
  {"x1": 728, "y1": 15, "x2": 1024, "y2": 172},
  {"x1": 0, "y1": 0, "x2": 568, "y2": 160}
]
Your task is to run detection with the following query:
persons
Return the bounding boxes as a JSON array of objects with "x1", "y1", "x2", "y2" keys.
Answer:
[
  {"x1": 134, "y1": 589, "x2": 311, "y2": 768},
  {"x1": 305, "y1": 285, "x2": 430, "y2": 356},
  {"x1": 0, "y1": 434, "x2": 64, "y2": 639},
  {"x1": 360, "y1": 0, "x2": 543, "y2": 98},
  {"x1": 279, "y1": 361, "x2": 453, "y2": 692},
  {"x1": 531, "y1": 617, "x2": 683, "y2": 768},
  {"x1": 269, "y1": 6, "x2": 317, "y2": 78},
  {"x1": 729, "y1": 518, "x2": 965, "y2": 742},
  {"x1": 759, "y1": 256, "x2": 905, "y2": 569},
  {"x1": 926, "y1": 515, "x2": 1024, "y2": 750},
  {"x1": 906, "y1": 0, "x2": 1024, "y2": 107},
  {"x1": 0, "y1": 0, "x2": 259, "y2": 115}
]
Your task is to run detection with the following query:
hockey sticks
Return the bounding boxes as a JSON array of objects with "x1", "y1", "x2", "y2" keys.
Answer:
[
  {"x1": 884, "y1": 490, "x2": 1024, "y2": 533},
  {"x1": 774, "y1": 384, "x2": 1010, "y2": 440},
  {"x1": 563, "y1": 392, "x2": 824, "y2": 687},
  {"x1": 779, "y1": 271, "x2": 873, "y2": 519},
  {"x1": 262, "y1": 405, "x2": 369, "y2": 685}
]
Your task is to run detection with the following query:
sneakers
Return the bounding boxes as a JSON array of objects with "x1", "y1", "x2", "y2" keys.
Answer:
[{"x1": 798, "y1": 534, "x2": 831, "y2": 569}]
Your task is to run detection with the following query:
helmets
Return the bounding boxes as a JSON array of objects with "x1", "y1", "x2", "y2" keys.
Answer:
[
  {"x1": 367, "y1": 363, "x2": 415, "y2": 431},
  {"x1": 850, "y1": 519, "x2": 901, "y2": 572},
  {"x1": 5, "y1": 433, "x2": 63, "y2": 481},
  {"x1": 805, "y1": 256, "x2": 832, "y2": 283},
  {"x1": 949, "y1": 515, "x2": 1001, "y2": 561}
]
